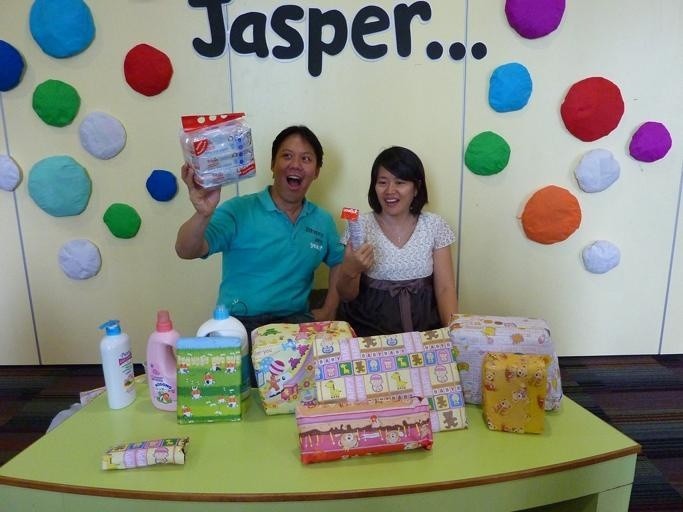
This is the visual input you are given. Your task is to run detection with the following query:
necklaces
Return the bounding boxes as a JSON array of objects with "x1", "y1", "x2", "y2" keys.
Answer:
[{"x1": 388, "y1": 220, "x2": 407, "y2": 241}]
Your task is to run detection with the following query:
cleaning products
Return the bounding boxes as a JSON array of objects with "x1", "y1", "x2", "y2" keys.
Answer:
[
  {"x1": 195, "y1": 304, "x2": 253, "y2": 416},
  {"x1": 97, "y1": 320, "x2": 137, "y2": 410},
  {"x1": 146, "y1": 311, "x2": 180, "y2": 411}
]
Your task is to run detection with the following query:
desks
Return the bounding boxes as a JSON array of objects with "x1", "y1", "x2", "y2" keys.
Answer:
[{"x1": 0, "y1": 374, "x2": 642, "y2": 512}]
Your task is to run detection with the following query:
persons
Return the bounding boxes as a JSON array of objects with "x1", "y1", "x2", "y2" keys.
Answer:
[
  {"x1": 336, "y1": 146, "x2": 458, "y2": 337},
  {"x1": 174, "y1": 124, "x2": 345, "y2": 387}
]
